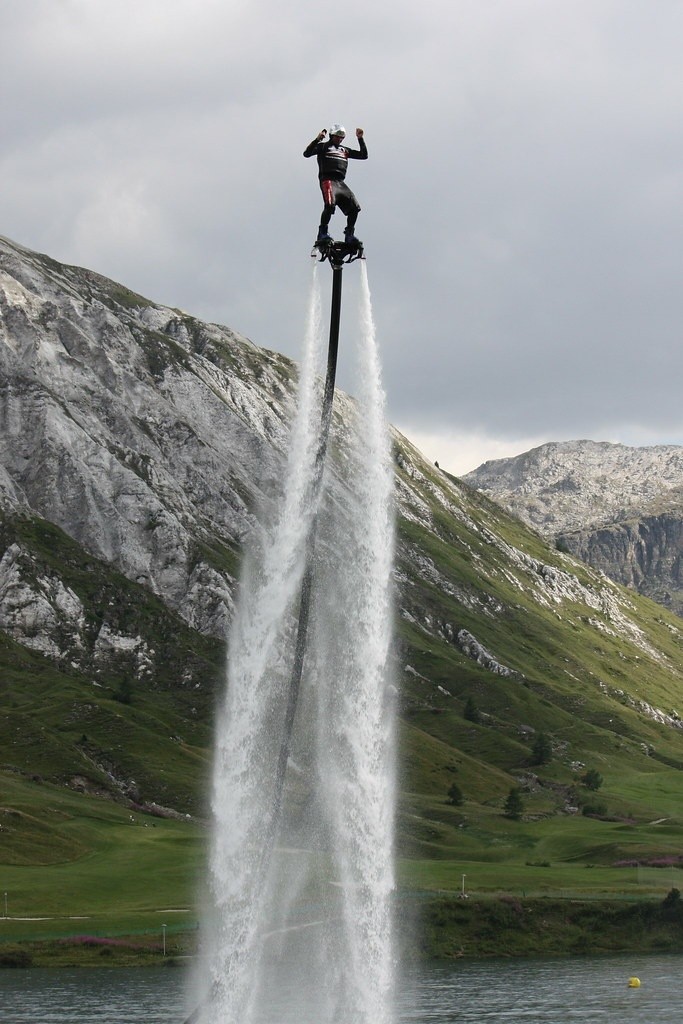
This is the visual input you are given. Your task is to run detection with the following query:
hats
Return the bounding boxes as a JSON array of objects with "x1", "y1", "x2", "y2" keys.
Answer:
[{"x1": 331, "y1": 123, "x2": 346, "y2": 138}]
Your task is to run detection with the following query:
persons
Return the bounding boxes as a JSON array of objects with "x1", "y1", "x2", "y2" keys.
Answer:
[{"x1": 304, "y1": 125, "x2": 368, "y2": 256}]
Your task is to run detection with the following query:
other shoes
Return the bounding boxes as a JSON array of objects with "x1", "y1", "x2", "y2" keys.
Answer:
[
  {"x1": 318, "y1": 232, "x2": 332, "y2": 239},
  {"x1": 345, "y1": 235, "x2": 362, "y2": 244}
]
